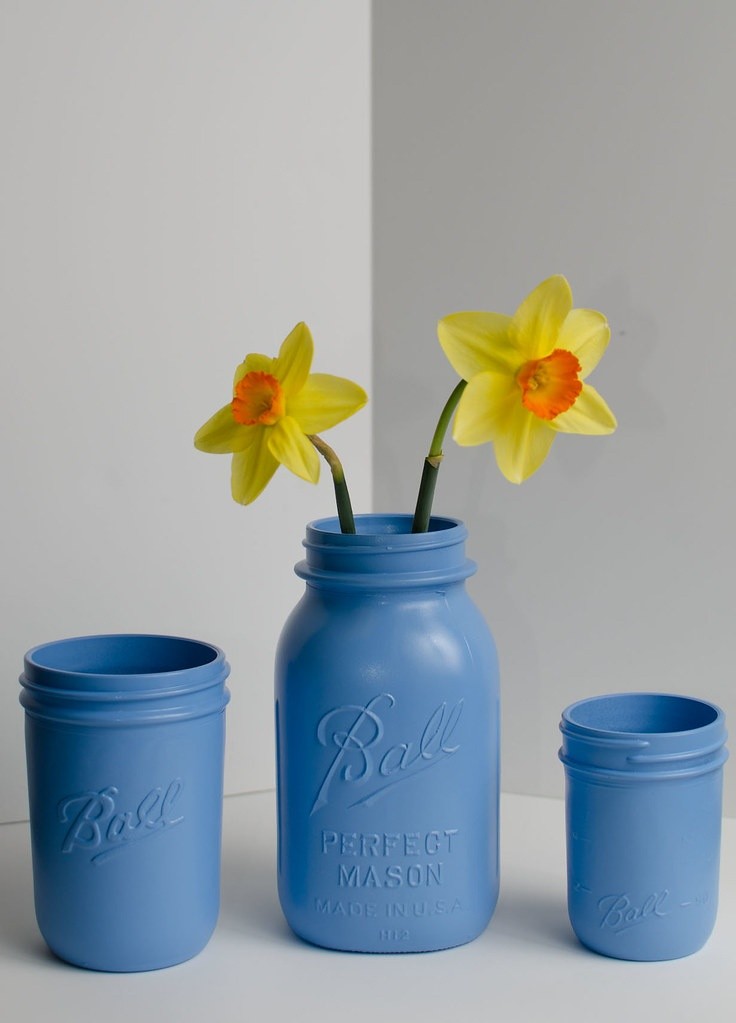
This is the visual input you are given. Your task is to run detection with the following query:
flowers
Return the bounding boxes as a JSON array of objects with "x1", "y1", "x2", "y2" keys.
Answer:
[
  {"x1": 411, "y1": 271, "x2": 619, "y2": 532},
  {"x1": 193, "y1": 321, "x2": 368, "y2": 533}
]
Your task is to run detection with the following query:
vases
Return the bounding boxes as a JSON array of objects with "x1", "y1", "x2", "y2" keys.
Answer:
[{"x1": 273, "y1": 513, "x2": 501, "y2": 954}]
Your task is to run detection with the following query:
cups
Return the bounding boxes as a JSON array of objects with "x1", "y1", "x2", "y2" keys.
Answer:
[
  {"x1": 16, "y1": 632, "x2": 229, "y2": 973},
  {"x1": 559, "y1": 691, "x2": 725, "y2": 963}
]
